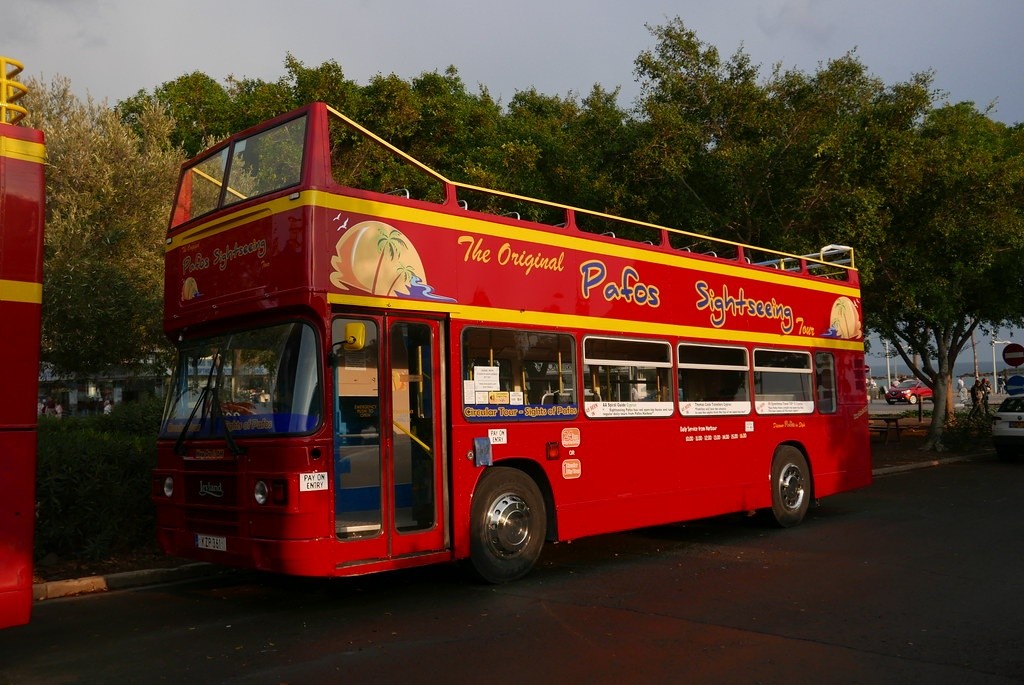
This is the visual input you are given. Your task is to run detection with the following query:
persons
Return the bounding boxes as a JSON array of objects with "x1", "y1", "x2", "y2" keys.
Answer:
[
  {"x1": 997, "y1": 373, "x2": 1008, "y2": 395},
  {"x1": 38, "y1": 393, "x2": 113, "y2": 417},
  {"x1": 967, "y1": 378, "x2": 988, "y2": 420},
  {"x1": 865, "y1": 374, "x2": 965, "y2": 397}
]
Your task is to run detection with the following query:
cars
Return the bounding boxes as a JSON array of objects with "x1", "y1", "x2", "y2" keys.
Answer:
[
  {"x1": 992, "y1": 394, "x2": 1024, "y2": 455},
  {"x1": 885, "y1": 379, "x2": 935, "y2": 405}
]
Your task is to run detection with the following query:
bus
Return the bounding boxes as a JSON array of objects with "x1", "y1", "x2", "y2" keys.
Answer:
[
  {"x1": 148, "y1": 100, "x2": 873, "y2": 579},
  {"x1": 0, "y1": 62, "x2": 50, "y2": 637}
]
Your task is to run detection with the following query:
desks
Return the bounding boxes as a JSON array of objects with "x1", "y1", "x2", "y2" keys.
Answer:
[{"x1": 869, "y1": 417, "x2": 902, "y2": 446}]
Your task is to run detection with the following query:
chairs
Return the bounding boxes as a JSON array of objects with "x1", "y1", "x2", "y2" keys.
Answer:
[{"x1": 389, "y1": 188, "x2": 831, "y2": 280}]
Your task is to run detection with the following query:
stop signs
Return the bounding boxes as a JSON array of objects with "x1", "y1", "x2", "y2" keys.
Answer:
[
  {"x1": 1002, "y1": 343, "x2": 1024, "y2": 366},
  {"x1": 865, "y1": 365, "x2": 869, "y2": 373}
]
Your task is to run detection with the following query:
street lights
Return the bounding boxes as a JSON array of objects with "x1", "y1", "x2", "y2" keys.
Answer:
[
  {"x1": 894, "y1": 345, "x2": 912, "y2": 381},
  {"x1": 992, "y1": 341, "x2": 1013, "y2": 394}
]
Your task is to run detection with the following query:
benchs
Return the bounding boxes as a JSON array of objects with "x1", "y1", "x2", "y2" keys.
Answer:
[{"x1": 869, "y1": 426, "x2": 908, "y2": 432}]
